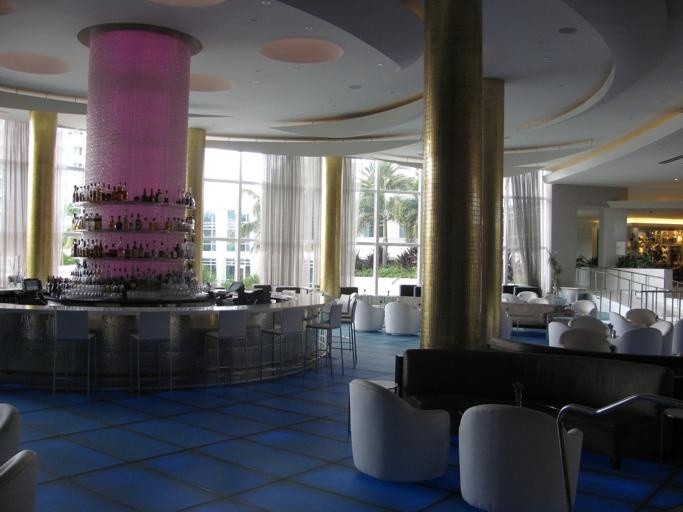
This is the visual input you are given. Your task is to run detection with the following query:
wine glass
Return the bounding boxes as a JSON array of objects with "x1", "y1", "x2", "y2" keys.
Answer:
[{"x1": 65, "y1": 283, "x2": 205, "y2": 302}]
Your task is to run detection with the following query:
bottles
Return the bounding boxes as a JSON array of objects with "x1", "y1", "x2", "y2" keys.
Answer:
[
  {"x1": 71, "y1": 181, "x2": 197, "y2": 283},
  {"x1": 42, "y1": 275, "x2": 67, "y2": 298}
]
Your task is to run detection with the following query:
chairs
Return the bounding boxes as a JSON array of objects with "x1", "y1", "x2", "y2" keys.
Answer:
[
  {"x1": 458, "y1": 403, "x2": 584, "y2": 512},
  {"x1": 0, "y1": 403, "x2": 20, "y2": 466},
  {"x1": 349, "y1": 378, "x2": 452, "y2": 481},
  {"x1": 1, "y1": 450, "x2": 39, "y2": 511}
]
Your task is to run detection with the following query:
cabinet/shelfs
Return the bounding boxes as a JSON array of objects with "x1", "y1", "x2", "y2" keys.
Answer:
[{"x1": 70, "y1": 200, "x2": 198, "y2": 263}]
